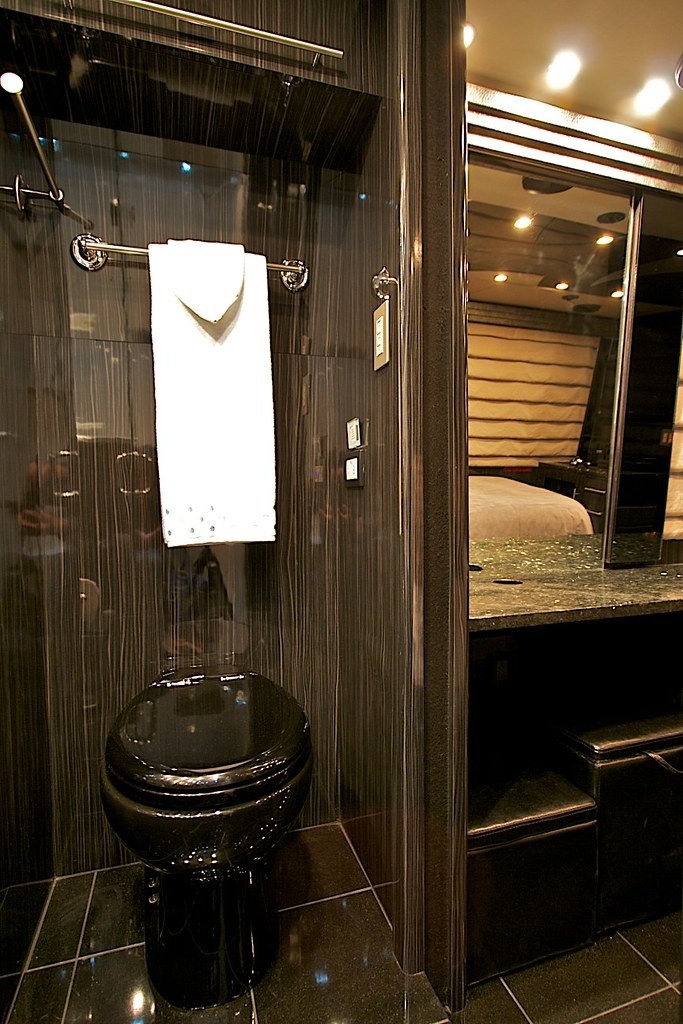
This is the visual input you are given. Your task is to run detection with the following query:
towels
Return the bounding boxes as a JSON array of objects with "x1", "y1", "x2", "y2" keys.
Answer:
[{"x1": 145, "y1": 236, "x2": 278, "y2": 549}]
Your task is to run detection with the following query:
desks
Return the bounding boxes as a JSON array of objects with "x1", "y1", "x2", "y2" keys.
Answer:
[{"x1": 538, "y1": 461, "x2": 612, "y2": 534}]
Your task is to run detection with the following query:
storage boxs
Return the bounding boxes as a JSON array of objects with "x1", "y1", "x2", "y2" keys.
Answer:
[{"x1": 468, "y1": 706, "x2": 683, "y2": 991}]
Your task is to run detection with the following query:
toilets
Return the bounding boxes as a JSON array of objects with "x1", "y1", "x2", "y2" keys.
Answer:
[
  {"x1": 97, "y1": 663, "x2": 316, "y2": 1013},
  {"x1": 152, "y1": 617, "x2": 262, "y2": 663}
]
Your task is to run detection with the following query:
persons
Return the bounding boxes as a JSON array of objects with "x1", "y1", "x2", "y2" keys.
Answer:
[{"x1": 17, "y1": 443, "x2": 232, "y2": 657}]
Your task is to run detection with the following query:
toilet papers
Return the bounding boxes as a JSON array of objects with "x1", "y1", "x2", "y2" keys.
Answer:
[{"x1": 76, "y1": 577, "x2": 107, "y2": 628}]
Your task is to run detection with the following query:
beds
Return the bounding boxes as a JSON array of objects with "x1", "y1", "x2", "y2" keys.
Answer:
[{"x1": 469, "y1": 474, "x2": 595, "y2": 537}]
